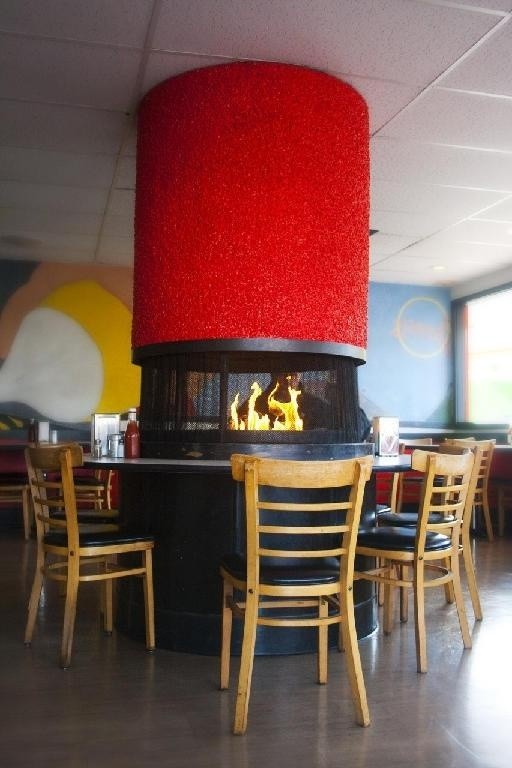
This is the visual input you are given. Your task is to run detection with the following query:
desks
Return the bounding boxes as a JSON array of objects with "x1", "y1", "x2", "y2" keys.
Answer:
[{"x1": 404, "y1": 444, "x2": 512, "y2": 537}]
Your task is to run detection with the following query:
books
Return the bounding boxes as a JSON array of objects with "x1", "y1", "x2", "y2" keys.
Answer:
[{"x1": 374, "y1": 416, "x2": 399, "y2": 455}]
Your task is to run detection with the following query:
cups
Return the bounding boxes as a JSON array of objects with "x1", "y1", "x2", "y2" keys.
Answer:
[
  {"x1": 50, "y1": 429, "x2": 57, "y2": 445},
  {"x1": 107, "y1": 432, "x2": 122, "y2": 458}
]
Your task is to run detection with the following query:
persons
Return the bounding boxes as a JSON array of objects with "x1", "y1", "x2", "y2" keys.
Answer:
[{"x1": 228, "y1": 371, "x2": 325, "y2": 431}]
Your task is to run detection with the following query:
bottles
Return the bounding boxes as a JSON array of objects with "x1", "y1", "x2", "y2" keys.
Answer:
[
  {"x1": 117, "y1": 439, "x2": 125, "y2": 458},
  {"x1": 125, "y1": 408, "x2": 140, "y2": 459},
  {"x1": 92, "y1": 439, "x2": 102, "y2": 460},
  {"x1": 27, "y1": 417, "x2": 35, "y2": 444}
]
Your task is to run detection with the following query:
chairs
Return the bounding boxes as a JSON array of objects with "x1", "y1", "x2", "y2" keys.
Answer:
[
  {"x1": 23, "y1": 446, "x2": 156, "y2": 669},
  {"x1": 219, "y1": 453, "x2": 374, "y2": 735},
  {"x1": 351, "y1": 452, "x2": 476, "y2": 675},
  {"x1": 377, "y1": 447, "x2": 483, "y2": 623},
  {"x1": 30, "y1": 440, "x2": 119, "y2": 597}
]
